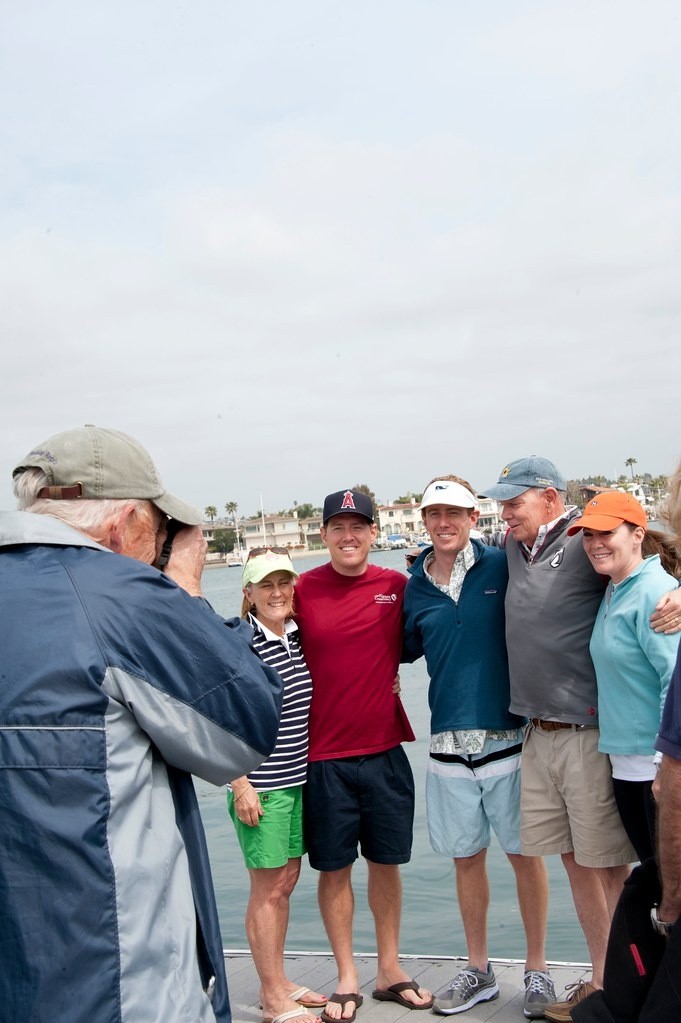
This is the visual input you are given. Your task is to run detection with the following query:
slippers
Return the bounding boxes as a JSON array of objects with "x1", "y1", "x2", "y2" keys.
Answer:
[
  {"x1": 322, "y1": 993, "x2": 363, "y2": 1023},
  {"x1": 263, "y1": 1004, "x2": 325, "y2": 1023},
  {"x1": 372, "y1": 979, "x2": 436, "y2": 1009},
  {"x1": 259, "y1": 987, "x2": 328, "y2": 1006}
]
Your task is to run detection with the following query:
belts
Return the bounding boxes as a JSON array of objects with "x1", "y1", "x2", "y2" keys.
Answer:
[{"x1": 532, "y1": 718, "x2": 572, "y2": 731}]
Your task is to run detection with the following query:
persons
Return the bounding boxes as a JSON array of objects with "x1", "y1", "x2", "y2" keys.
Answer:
[
  {"x1": 401, "y1": 455, "x2": 681, "y2": 1023},
  {"x1": 227, "y1": 548, "x2": 329, "y2": 1023},
  {"x1": 290, "y1": 490, "x2": 435, "y2": 1023},
  {"x1": 0, "y1": 424, "x2": 284, "y2": 1023},
  {"x1": 397, "y1": 475, "x2": 558, "y2": 1020},
  {"x1": 567, "y1": 462, "x2": 681, "y2": 1023}
]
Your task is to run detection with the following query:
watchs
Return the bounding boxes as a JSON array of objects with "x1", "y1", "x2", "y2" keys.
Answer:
[{"x1": 650, "y1": 902, "x2": 676, "y2": 935}]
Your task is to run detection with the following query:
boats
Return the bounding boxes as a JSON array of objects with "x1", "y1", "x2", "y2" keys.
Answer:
[
  {"x1": 387, "y1": 534, "x2": 407, "y2": 550},
  {"x1": 418, "y1": 541, "x2": 434, "y2": 548}
]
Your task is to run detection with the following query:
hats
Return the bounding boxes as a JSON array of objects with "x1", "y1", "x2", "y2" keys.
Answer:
[
  {"x1": 243, "y1": 549, "x2": 300, "y2": 588},
  {"x1": 323, "y1": 491, "x2": 374, "y2": 527},
  {"x1": 418, "y1": 481, "x2": 476, "y2": 510},
  {"x1": 568, "y1": 492, "x2": 647, "y2": 536},
  {"x1": 13, "y1": 424, "x2": 203, "y2": 526},
  {"x1": 478, "y1": 455, "x2": 567, "y2": 501}
]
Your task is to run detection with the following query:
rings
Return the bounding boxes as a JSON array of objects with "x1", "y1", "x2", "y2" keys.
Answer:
[
  {"x1": 239, "y1": 816, "x2": 244, "y2": 821},
  {"x1": 675, "y1": 617, "x2": 679, "y2": 625}
]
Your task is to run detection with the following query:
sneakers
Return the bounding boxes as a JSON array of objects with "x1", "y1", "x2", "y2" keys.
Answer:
[
  {"x1": 432, "y1": 962, "x2": 499, "y2": 1014},
  {"x1": 523, "y1": 968, "x2": 557, "y2": 1017},
  {"x1": 545, "y1": 980, "x2": 599, "y2": 1023}
]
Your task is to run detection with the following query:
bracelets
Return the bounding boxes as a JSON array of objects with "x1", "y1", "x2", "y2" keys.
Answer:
[{"x1": 233, "y1": 783, "x2": 252, "y2": 802}]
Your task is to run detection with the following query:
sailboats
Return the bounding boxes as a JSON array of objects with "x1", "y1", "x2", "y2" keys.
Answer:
[{"x1": 227, "y1": 509, "x2": 245, "y2": 567}]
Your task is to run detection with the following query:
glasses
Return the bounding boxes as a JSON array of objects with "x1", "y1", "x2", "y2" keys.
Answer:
[{"x1": 242, "y1": 548, "x2": 290, "y2": 578}]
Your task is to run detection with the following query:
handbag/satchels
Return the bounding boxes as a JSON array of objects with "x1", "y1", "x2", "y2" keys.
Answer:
[{"x1": 571, "y1": 861, "x2": 681, "y2": 1023}]
